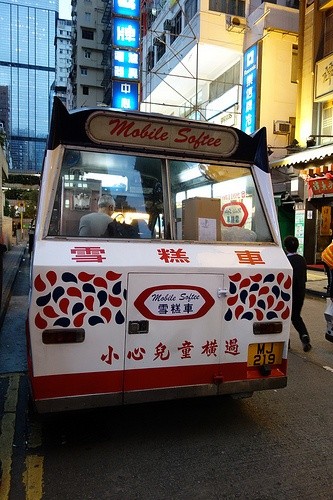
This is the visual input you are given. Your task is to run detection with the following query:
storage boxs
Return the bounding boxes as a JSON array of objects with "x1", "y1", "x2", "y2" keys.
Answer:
[{"x1": 181, "y1": 197, "x2": 222, "y2": 241}]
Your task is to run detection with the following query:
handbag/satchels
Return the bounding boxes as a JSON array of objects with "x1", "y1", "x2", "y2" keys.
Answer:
[{"x1": 104, "y1": 215, "x2": 136, "y2": 238}]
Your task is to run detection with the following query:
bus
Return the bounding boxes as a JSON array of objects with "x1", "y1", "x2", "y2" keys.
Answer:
[{"x1": 23, "y1": 96, "x2": 294, "y2": 417}]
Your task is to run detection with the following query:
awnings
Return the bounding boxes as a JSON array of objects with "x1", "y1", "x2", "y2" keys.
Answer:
[{"x1": 268, "y1": 142, "x2": 333, "y2": 169}]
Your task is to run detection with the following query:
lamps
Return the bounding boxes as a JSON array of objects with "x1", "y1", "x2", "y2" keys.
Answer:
[
  {"x1": 307, "y1": 135, "x2": 333, "y2": 147},
  {"x1": 267, "y1": 145, "x2": 290, "y2": 156}
]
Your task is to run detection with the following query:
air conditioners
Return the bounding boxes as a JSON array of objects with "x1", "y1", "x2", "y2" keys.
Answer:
[
  {"x1": 273, "y1": 120, "x2": 292, "y2": 135},
  {"x1": 163, "y1": 20, "x2": 175, "y2": 30},
  {"x1": 147, "y1": 46, "x2": 154, "y2": 54},
  {"x1": 153, "y1": 37, "x2": 164, "y2": 46},
  {"x1": 226, "y1": 16, "x2": 247, "y2": 33}
]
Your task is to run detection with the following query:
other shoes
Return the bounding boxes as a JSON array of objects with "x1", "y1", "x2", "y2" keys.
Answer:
[{"x1": 302, "y1": 334, "x2": 311, "y2": 352}]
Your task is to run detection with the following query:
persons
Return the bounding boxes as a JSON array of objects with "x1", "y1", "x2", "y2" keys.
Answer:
[
  {"x1": 283, "y1": 236, "x2": 311, "y2": 352},
  {"x1": 79, "y1": 195, "x2": 116, "y2": 236},
  {"x1": 12, "y1": 221, "x2": 17, "y2": 237},
  {"x1": 221, "y1": 223, "x2": 257, "y2": 242},
  {"x1": 321, "y1": 243, "x2": 333, "y2": 301}
]
